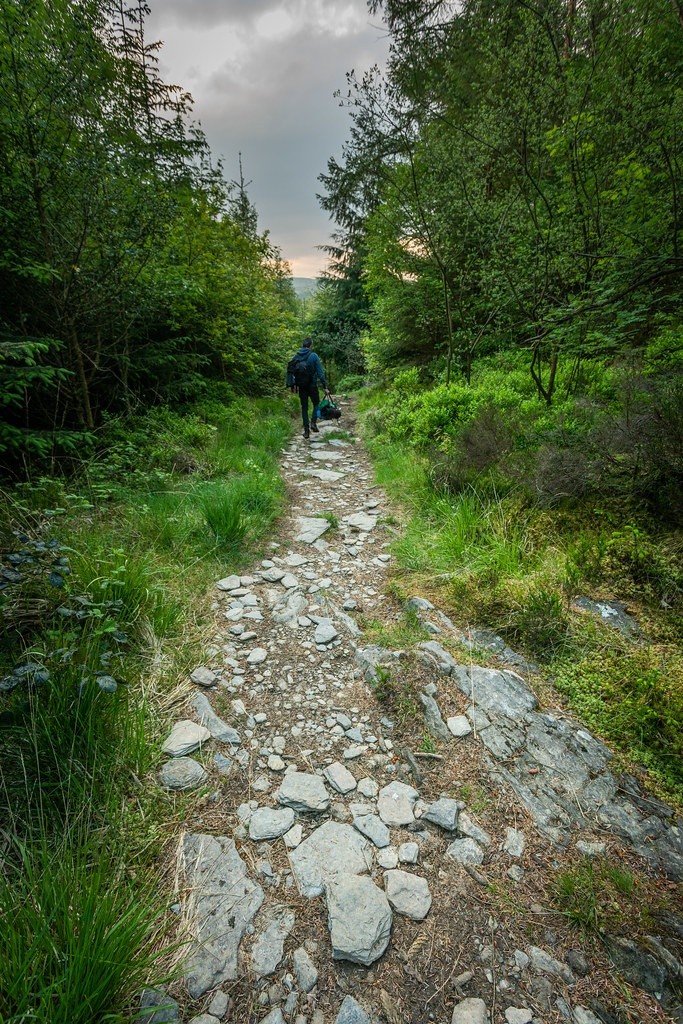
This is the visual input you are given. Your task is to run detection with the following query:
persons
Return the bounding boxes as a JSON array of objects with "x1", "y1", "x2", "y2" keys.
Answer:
[{"x1": 290, "y1": 337, "x2": 330, "y2": 438}]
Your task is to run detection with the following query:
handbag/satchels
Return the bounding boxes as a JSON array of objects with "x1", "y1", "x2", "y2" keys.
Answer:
[{"x1": 317, "y1": 393, "x2": 341, "y2": 420}]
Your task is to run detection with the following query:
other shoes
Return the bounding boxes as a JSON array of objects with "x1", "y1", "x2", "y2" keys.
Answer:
[
  {"x1": 311, "y1": 422, "x2": 319, "y2": 433},
  {"x1": 303, "y1": 432, "x2": 309, "y2": 439}
]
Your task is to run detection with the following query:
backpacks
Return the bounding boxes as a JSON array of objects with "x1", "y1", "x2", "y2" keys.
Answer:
[{"x1": 294, "y1": 352, "x2": 311, "y2": 393}]
